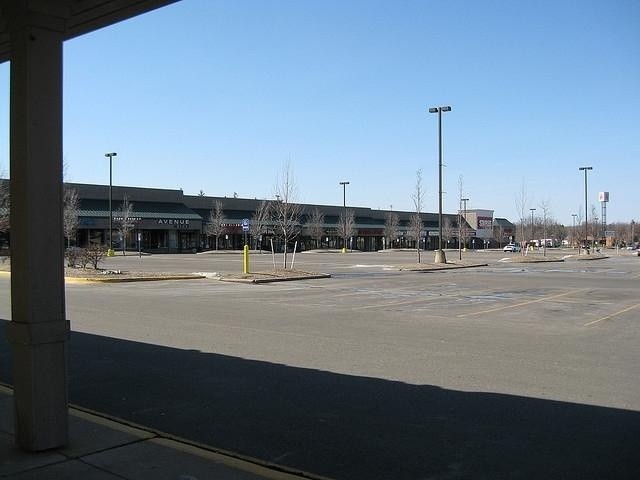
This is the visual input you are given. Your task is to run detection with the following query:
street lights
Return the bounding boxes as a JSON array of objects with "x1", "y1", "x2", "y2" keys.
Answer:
[
  {"x1": 579, "y1": 167, "x2": 592, "y2": 255},
  {"x1": 429, "y1": 106, "x2": 451, "y2": 263},
  {"x1": 572, "y1": 215, "x2": 577, "y2": 245},
  {"x1": 461, "y1": 199, "x2": 469, "y2": 252},
  {"x1": 105, "y1": 153, "x2": 117, "y2": 256},
  {"x1": 530, "y1": 209, "x2": 536, "y2": 251},
  {"x1": 340, "y1": 182, "x2": 349, "y2": 253}
]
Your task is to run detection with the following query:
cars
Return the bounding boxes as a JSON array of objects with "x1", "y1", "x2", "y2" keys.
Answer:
[
  {"x1": 503, "y1": 244, "x2": 521, "y2": 252},
  {"x1": 627, "y1": 246, "x2": 634, "y2": 250}
]
